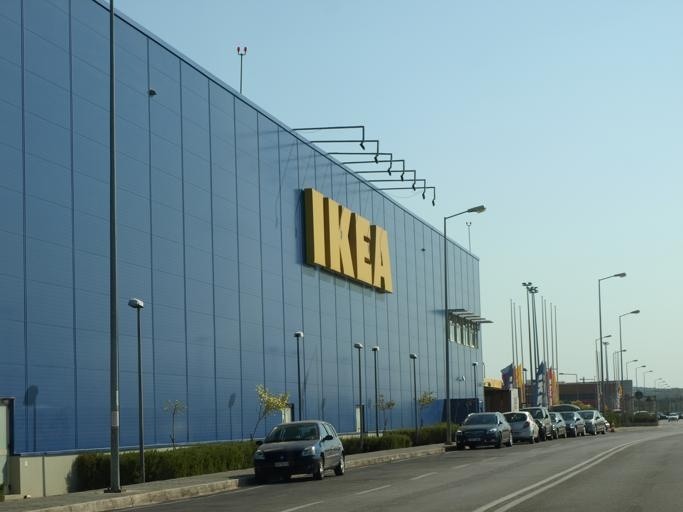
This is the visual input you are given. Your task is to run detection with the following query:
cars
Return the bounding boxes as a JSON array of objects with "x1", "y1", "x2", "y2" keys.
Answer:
[
  {"x1": 548, "y1": 412, "x2": 567, "y2": 439},
  {"x1": 559, "y1": 412, "x2": 586, "y2": 437},
  {"x1": 502, "y1": 411, "x2": 539, "y2": 444},
  {"x1": 253, "y1": 420, "x2": 345, "y2": 483},
  {"x1": 575, "y1": 410, "x2": 609, "y2": 435},
  {"x1": 612, "y1": 408, "x2": 682, "y2": 423},
  {"x1": 454, "y1": 412, "x2": 513, "y2": 450}
]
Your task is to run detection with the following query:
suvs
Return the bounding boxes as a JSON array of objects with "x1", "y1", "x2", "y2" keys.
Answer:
[{"x1": 520, "y1": 408, "x2": 553, "y2": 442}]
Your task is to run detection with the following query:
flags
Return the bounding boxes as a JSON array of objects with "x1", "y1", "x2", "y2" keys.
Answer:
[
  {"x1": 605, "y1": 383, "x2": 624, "y2": 401},
  {"x1": 499, "y1": 361, "x2": 560, "y2": 407}
]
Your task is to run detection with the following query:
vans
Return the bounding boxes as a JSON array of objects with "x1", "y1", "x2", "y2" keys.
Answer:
[{"x1": 550, "y1": 404, "x2": 579, "y2": 412}]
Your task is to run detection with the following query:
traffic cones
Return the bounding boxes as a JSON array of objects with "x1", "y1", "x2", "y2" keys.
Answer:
[{"x1": 609, "y1": 421, "x2": 615, "y2": 431}]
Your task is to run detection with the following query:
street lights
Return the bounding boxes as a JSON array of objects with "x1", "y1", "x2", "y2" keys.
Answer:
[
  {"x1": 443, "y1": 204, "x2": 486, "y2": 444},
  {"x1": 409, "y1": 352, "x2": 419, "y2": 446},
  {"x1": 593, "y1": 273, "x2": 672, "y2": 424},
  {"x1": 471, "y1": 362, "x2": 478, "y2": 413},
  {"x1": 559, "y1": 372, "x2": 578, "y2": 401},
  {"x1": 294, "y1": 331, "x2": 303, "y2": 421},
  {"x1": 371, "y1": 346, "x2": 380, "y2": 438},
  {"x1": 354, "y1": 343, "x2": 365, "y2": 449},
  {"x1": 522, "y1": 282, "x2": 541, "y2": 406},
  {"x1": 128, "y1": 297, "x2": 145, "y2": 484}
]
